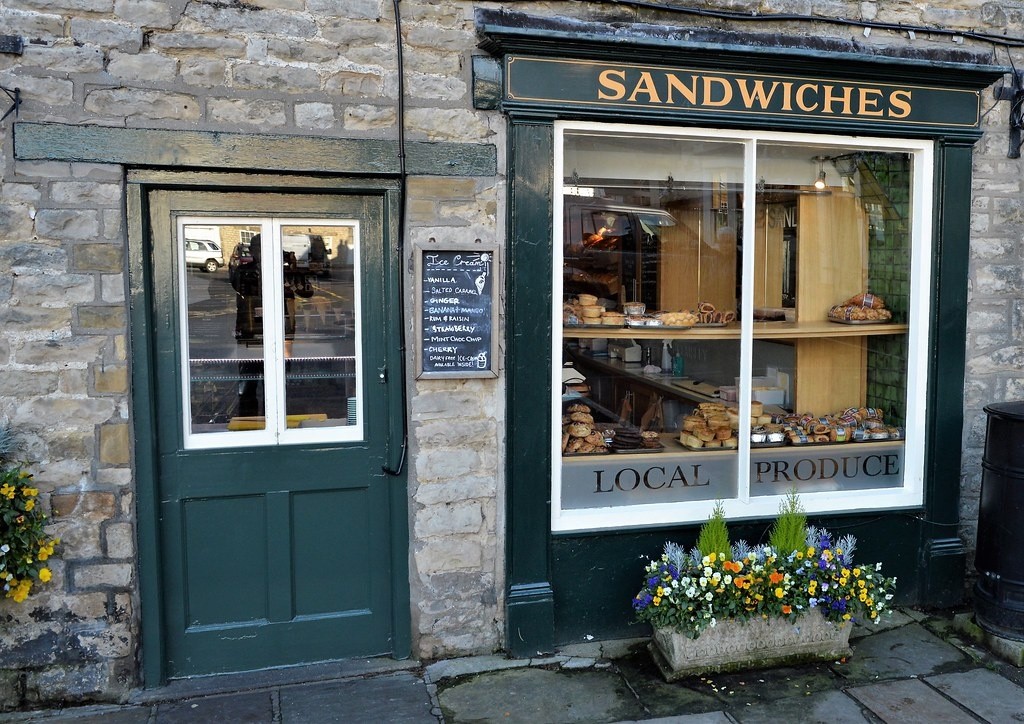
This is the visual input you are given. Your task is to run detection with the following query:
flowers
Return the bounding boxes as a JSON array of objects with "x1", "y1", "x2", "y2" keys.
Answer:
[{"x1": 627, "y1": 484, "x2": 900, "y2": 640}]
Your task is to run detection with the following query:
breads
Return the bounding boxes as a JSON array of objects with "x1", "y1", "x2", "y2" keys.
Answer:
[
  {"x1": 679, "y1": 400, "x2": 905, "y2": 449},
  {"x1": 829, "y1": 293, "x2": 891, "y2": 320},
  {"x1": 563, "y1": 294, "x2": 734, "y2": 326},
  {"x1": 562, "y1": 403, "x2": 661, "y2": 453}
]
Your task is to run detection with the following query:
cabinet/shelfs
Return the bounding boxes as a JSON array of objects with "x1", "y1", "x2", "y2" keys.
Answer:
[
  {"x1": 562, "y1": 349, "x2": 700, "y2": 431},
  {"x1": 655, "y1": 184, "x2": 868, "y2": 450},
  {"x1": 561, "y1": 315, "x2": 910, "y2": 463}
]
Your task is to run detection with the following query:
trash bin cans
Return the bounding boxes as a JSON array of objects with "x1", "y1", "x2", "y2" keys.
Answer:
[{"x1": 974, "y1": 402, "x2": 1024, "y2": 643}]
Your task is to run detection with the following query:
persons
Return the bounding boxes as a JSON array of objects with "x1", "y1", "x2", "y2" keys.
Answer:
[{"x1": 231, "y1": 232, "x2": 317, "y2": 434}]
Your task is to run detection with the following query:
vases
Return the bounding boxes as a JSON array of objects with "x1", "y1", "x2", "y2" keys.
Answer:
[{"x1": 646, "y1": 604, "x2": 853, "y2": 684}]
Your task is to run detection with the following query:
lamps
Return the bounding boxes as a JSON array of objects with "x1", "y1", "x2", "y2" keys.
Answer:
[{"x1": 811, "y1": 155, "x2": 831, "y2": 189}]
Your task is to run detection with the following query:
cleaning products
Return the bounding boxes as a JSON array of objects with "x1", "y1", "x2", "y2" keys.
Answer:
[
  {"x1": 661, "y1": 339, "x2": 672, "y2": 372},
  {"x1": 672, "y1": 350, "x2": 684, "y2": 377}
]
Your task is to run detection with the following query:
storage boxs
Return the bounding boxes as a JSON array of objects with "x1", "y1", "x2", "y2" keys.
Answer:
[
  {"x1": 733, "y1": 376, "x2": 775, "y2": 403},
  {"x1": 751, "y1": 387, "x2": 786, "y2": 405},
  {"x1": 719, "y1": 386, "x2": 737, "y2": 402}
]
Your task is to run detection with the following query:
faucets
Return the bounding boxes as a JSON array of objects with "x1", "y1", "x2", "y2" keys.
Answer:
[{"x1": 647, "y1": 346, "x2": 651, "y2": 365}]
